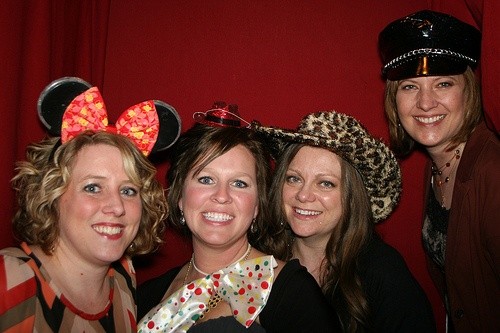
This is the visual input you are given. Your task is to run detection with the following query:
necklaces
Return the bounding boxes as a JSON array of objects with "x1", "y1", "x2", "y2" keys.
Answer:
[
  {"x1": 183, "y1": 245, "x2": 252, "y2": 285},
  {"x1": 431, "y1": 146, "x2": 463, "y2": 210}
]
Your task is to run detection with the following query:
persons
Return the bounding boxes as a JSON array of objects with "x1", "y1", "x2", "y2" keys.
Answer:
[
  {"x1": 250, "y1": 110, "x2": 438, "y2": 333},
  {"x1": 137, "y1": 100, "x2": 342, "y2": 333},
  {"x1": 374, "y1": 10, "x2": 500, "y2": 333},
  {"x1": 0, "y1": 77, "x2": 182, "y2": 333}
]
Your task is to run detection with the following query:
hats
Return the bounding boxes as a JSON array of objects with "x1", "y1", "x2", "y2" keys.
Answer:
[
  {"x1": 250, "y1": 110, "x2": 403, "y2": 223},
  {"x1": 378, "y1": 9, "x2": 482, "y2": 80}
]
